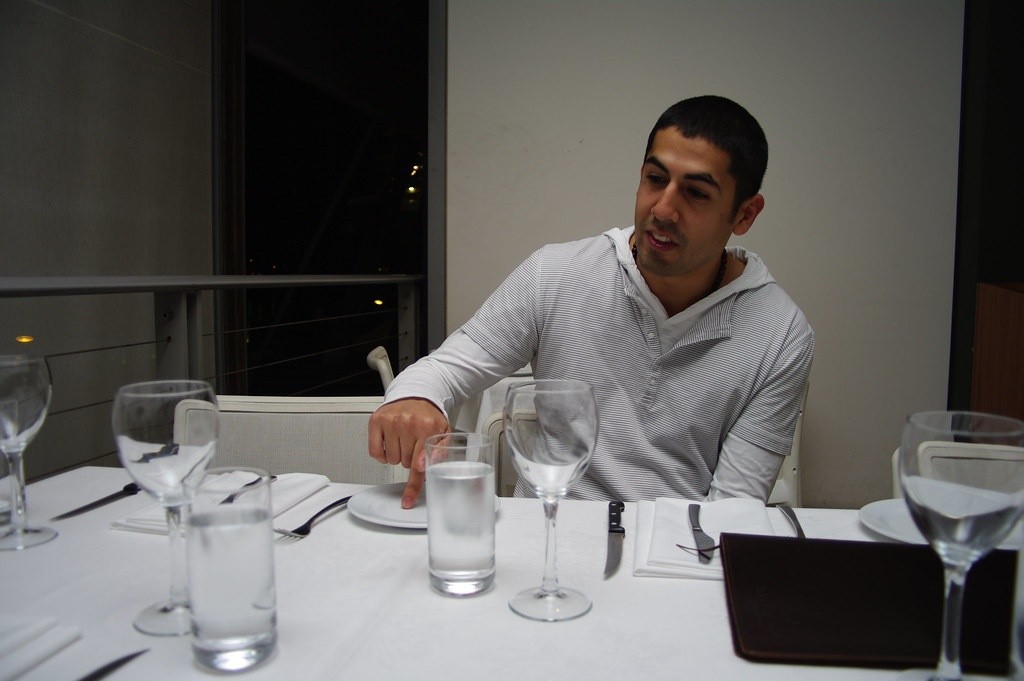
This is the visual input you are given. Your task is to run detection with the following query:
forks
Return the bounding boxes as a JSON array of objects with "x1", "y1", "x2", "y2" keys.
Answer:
[{"x1": 271, "y1": 495, "x2": 351, "y2": 537}]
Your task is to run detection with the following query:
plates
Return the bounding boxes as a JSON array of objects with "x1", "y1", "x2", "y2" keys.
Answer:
[
  {"x1": 346, "y1": 481, "x2": 428, "y2": 530},
  {"x1": 858, "y1": 498, "x2": 1024, "y2": 549}
]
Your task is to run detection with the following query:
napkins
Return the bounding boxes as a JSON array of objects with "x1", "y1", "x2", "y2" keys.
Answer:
[
  {"x1": 111, "y1": 471, "x2": 330, "y2": 538},
  {"x1": 632, "y1": 496, "x2": 773, "y2": 580}
]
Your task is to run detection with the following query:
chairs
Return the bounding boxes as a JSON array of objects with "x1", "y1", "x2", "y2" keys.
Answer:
[
  {"x1": 917, "y1": 440, "x2": 1024, "y2": 494},
  {"x1": 483, "y1": 410, "x2": 540, "y2": 499},
  {"x1": 171, "y1": 396, "x2": 455, "y2": 486}
]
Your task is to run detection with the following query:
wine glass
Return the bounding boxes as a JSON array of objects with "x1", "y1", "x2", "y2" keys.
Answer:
[
  {"x1": 0, "y1": 354, "x2": 58, "y2": 551},
  {"x1": 112, "y1": 379, "x2": 222, "y2": 639},
  {"x1": 502, "y1": 378, "x2": 600, "y2": 622},
  {"x1": 899, "y1": 410, "x2": 1024, "y2": 681}
]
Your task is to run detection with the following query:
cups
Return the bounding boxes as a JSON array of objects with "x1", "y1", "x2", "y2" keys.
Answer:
[
  {"x1": 425, "y1": 432, "x2": 496, "y2": 597},
  {"x1": 179, "y1": 467, "x2": 280, "y2": 675},
  {"x1": 1, "y1": 398, "x2": 19, "y2": 537}
]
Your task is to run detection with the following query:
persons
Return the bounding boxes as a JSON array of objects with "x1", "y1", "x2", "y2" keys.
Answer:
[{"x1": 367, "y1": 96, "x2": 816, "y2": 510}]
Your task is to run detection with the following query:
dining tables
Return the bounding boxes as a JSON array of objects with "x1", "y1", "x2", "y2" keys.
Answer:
[{"x1": 0, "y1": 461, "x2": 1024, "y2": 681}]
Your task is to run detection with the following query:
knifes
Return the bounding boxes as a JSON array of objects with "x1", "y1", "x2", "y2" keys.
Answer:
[
  {"x1": 603, "y1": 501, "x2": 625, "y2": 574},
  {"x1": 49, "y1": 481, "x2": 141, "y2": 521},
  {"x1": 688, "y1": 503, "x2": 715, "y2": 564}
]
